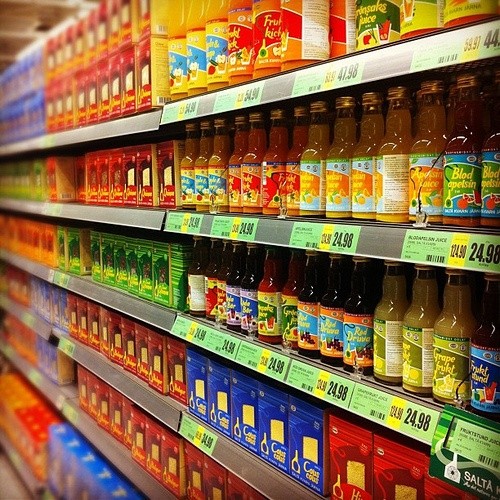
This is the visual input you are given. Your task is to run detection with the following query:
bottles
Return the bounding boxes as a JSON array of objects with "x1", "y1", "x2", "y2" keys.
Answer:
[{"x1": 163, "y1": 0, "x2": 500, "y2": 420}]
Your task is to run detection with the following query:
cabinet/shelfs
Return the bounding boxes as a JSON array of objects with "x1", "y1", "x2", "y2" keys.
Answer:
[{"x1": 1, "y1": 15, "x2": 499, "y2": 500}]
